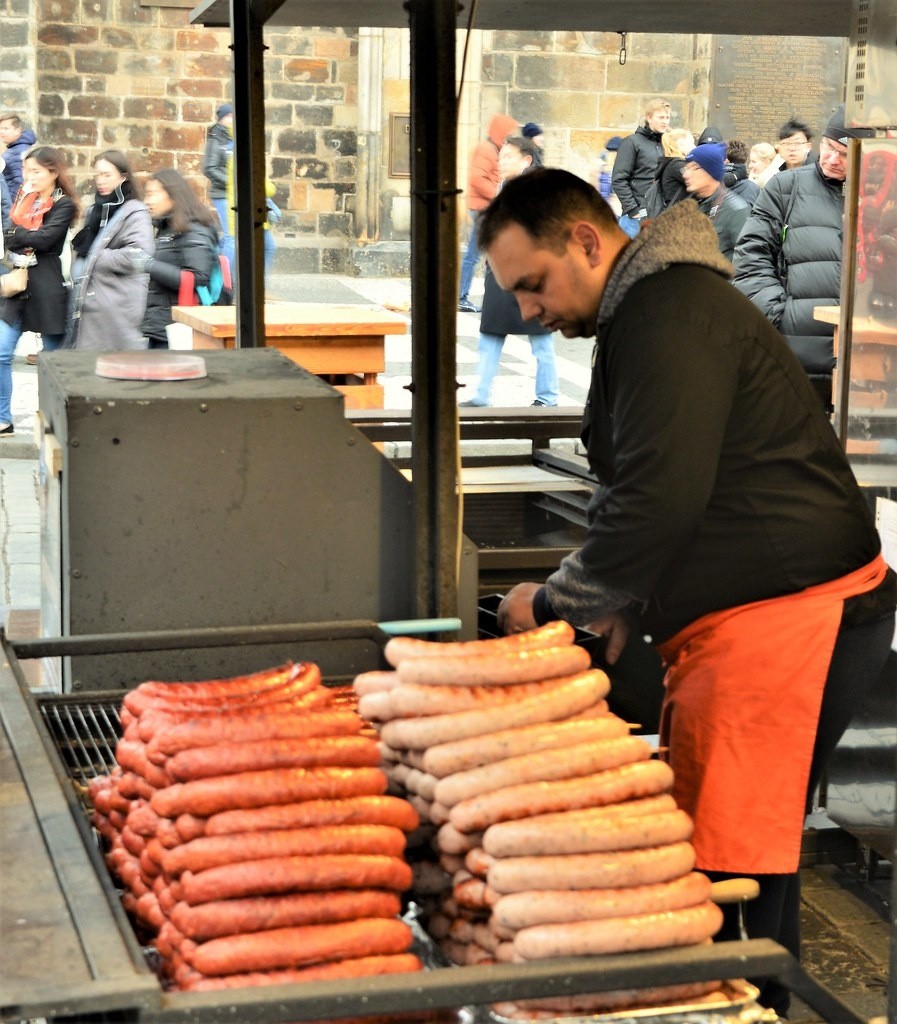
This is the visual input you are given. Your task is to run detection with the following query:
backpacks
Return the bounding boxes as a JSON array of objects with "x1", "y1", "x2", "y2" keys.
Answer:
[{"x1": 642, "y1": 180, "x2": 685, "y2": 219}]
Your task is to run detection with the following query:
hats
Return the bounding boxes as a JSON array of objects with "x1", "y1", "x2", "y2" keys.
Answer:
[
  {"x1": 522, "y1": 122, "x2": 543, "y2": 137},
  {"x1": 606, "y1": 137, "x2": 622, "y2": 150},
  {"x1": 686, "y1": 142, "x2": 727, "y2": 181},
  {"x1": 217, "y1": 103, "x2": 233, "y2": 120},
  {"x1": 822, "y1": 102, "x2": 876, "y2": 148}
]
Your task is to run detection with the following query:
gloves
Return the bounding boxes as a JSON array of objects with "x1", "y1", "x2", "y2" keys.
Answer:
[{"x1": 130, "y1": 248, "x2": 154, "y2": 273}]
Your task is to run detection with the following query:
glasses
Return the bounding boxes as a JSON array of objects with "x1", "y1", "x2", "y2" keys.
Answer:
[
  {"x1": 680, "y1": 167, "x2": 704, "y2": 175},
  {"x1": 780, "y1": 142, "x2": 808, "y2": 147},
  {"x1": 824, "y1": 138, "x2": 847, "y2": 159}
]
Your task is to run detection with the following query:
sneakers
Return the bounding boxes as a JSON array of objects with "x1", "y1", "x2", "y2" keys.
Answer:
[{"x1": 458, "y1": 299, "x2": 482, "y2": 312}]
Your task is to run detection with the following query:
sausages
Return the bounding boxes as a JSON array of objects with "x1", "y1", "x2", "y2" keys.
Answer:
[
  {"x1": 87, "y1": 662, "x2": 425, "y2": 996},
  {"x1": 354, "y1": 618, "x2": 728, "y2": 1015}
]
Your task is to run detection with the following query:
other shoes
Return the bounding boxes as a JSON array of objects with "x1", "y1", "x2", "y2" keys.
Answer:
[
  {"x1": 0, "y1": 424, "x2": 15, "y2": 437},
  {"x1": 458, "y1": 401, "x2": 477, "y2": 407},
  {"x1": 529, "y1": 400, "x2": 559, "y2": 407},
  {"x1": 26, "y1": 354, "x2": 38, "y2": 364}
]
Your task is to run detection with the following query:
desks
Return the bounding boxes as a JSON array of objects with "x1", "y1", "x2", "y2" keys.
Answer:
[{"x1": 171, "y1": 303, "x2": 407, "y2": 450}]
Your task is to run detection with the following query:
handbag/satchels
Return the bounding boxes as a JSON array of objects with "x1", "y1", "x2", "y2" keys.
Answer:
[
  {"x1": 1, "y1": 268, "x2": 29, "y2": 298},
  {"x1": 178, "y1": 255, "x2": 234, "y2": 305}
]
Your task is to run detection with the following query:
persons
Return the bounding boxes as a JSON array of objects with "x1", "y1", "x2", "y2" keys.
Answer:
[
  {"x1": 449, "y1": 99, "x2": 874, "y2": 467},
  {"x1": 1, "y1": 103, "x2": 284, "y2": 445},
  {"x1": 478, "y1": 166, "x2": 897, "y2": 1024}
]
